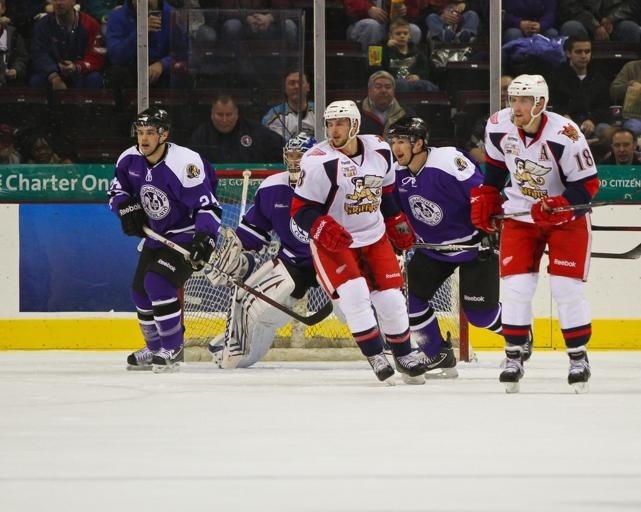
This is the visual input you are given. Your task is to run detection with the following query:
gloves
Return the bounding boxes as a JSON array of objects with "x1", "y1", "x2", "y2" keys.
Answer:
[
  {"x1": 118, "y1": 198, "x2": 151, "y2": 240},
  {"x1": 468, "y1": 184, "x2": 506, "y2": 234},
  {"x1": 386, "y1": 210, "x2": 417, "y2": 252},
  {"x1": 187, "y1": 230, "x2": 215, "y2": 272},
  {"x1": 531, "y1": 193, "x2": 574, "y2": 230},
  {"x1": 309, "y1": 214, "x2": 356, "y2": 255}
]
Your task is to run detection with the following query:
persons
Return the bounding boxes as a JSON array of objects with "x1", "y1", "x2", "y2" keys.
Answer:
[
  {"x1": 469, "y1": 74, "x2": 600, "y2": 383},
  {"x1": 385, "y1": 115, "x2": 533, "y2": 371},
  {"x1": 208, "y1": 135, "x2": 317, "y2": 369},
  {"x1": 291, "y1": 101, "x2": 428, "y2": 380},
  {"x1": 108, "y1": 107, "x2": 221, "y2": 365},
  {"x1": 343, "y1": 1, "x2": 641, "y2": 166},
  {"x1": 1, "y1": 2, "x2": 315, "y2": 163}
]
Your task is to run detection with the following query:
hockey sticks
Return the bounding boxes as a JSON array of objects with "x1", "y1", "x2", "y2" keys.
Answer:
[
  {"x1": 143, "y1": 226, "x2": 333, "y2": 326},
  {"x1": 215, "y1": 170, "x2": 251, "y2": 370},
  {"x1": 540, "y1": 242, "x2": 641, "y2": 260},
  {"x1": 491, "y1": 201, "x2": 607, "y2": 220}
]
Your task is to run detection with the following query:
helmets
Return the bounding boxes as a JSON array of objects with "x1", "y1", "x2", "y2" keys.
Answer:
[
  {"x1": 321, "y1": 98, "x2": 361, "y2": 148},
  {"x1": 128, "y1": 106, "x2": 175, "y2": 158},
  {"x1": 282, "y1": 132, "x2": 318, "y2": 170},
  {"x1": 385, "y1": 114, "x2": 431, "y2": 167},
  {"x1": 504, "y1": 73, "x2": 549, "y2": 130}
]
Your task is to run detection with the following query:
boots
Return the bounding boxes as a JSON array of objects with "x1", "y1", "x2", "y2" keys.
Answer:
[
  {"x1": 367, "y1": 351, "x2": 396, "y2": 381},
  {"x1": 567, "y1": 347, "x2": 593, "y2": 385},
  {"x1": 418, "y1": 345, "x2": 458, "y2": 372},
  {"x1": 392, "y1": 354, "x2": 426, "y2": 379},
  {"x1": 151, "y1": 345, "x2": 184, "y2": 365},
  {"x1": 126, "y1": 346, "x2": 162, "y2": 368},
  {"x1": 498, "y1": 343, "x2": 526, "y2": 383}
]
